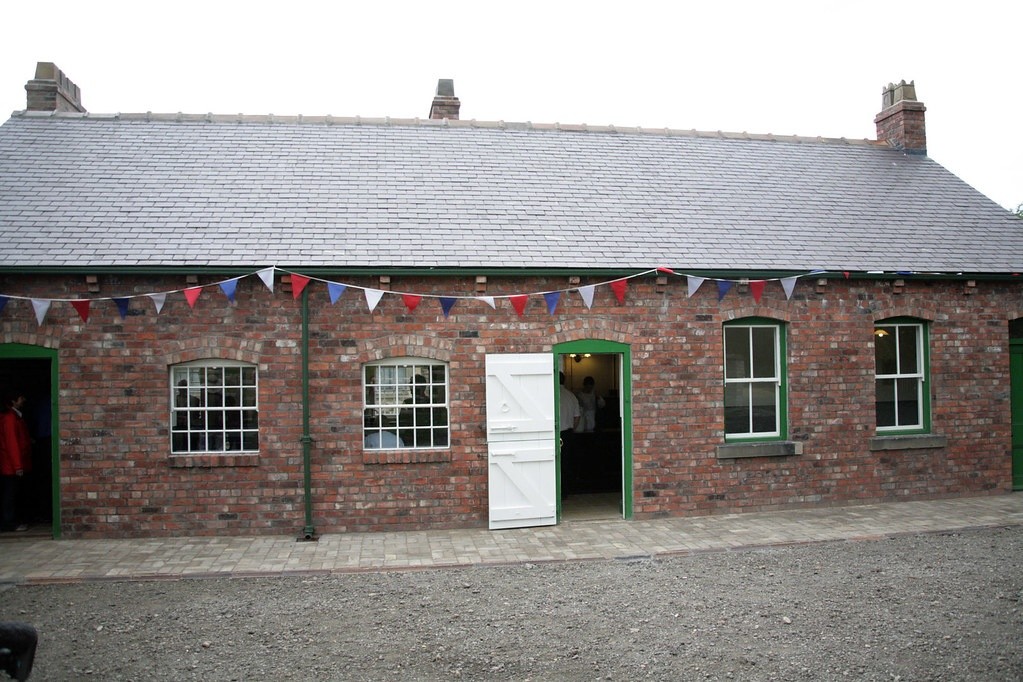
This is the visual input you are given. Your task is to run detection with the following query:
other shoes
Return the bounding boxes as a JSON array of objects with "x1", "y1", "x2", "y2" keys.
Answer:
[{"x1": 15, "y1": 524, "x2": 28, "y2": 531}]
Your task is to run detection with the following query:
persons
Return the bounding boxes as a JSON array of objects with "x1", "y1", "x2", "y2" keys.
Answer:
[
  {"x1": 198, "y1": 374, "x2": 248, "y2": 450},
  {"x1": 577, "y1": 376, "x2": 605, "y2": 431},
  {"x1": 0, "y1": 385, "x2": 50, "y2": 531},
  {"x1": 365, "y1": 415, "x2": 404, "y2": 448},
  {"x1": 397, "y1": 375, "x2": 441, "y2": 446},
  {"x1": 559, "y1": 372, "x2": 581, "y2": 495},
  {"x1": 177, "y1": 380, "x2": 200, "y2": 450}
]
